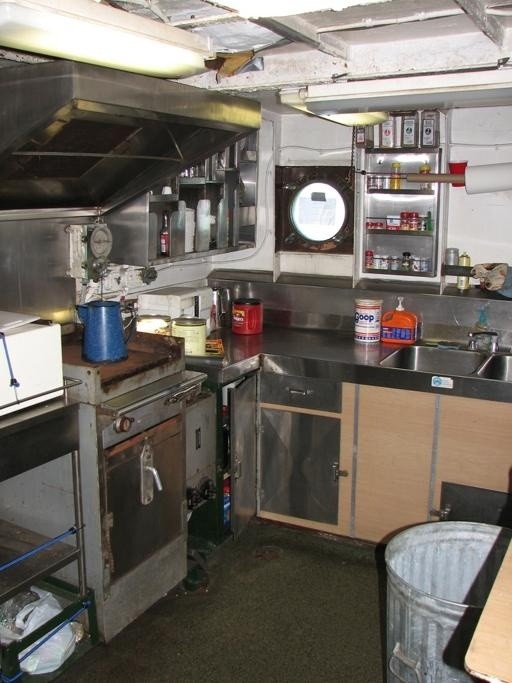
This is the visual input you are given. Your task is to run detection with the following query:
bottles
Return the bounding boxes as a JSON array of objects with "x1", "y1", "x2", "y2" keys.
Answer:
[
  {"x1": 401, "y1": 112, "x2": 419, "y2": 148},
  {"x1": 446, "y1": 247, "x2": 458, "y2": 267},
  {"x1": 456, "y1": 252, "x2": 471, "y2": 291},
  {"x1": 420, "y1": 109, "x2": 440, "y2": 148},
  {"x1": 159, "y1": 209, "x2": 171, "y2": 257},
  {"x1": 419, "y1": 163, "x2": 432, "y2": 191},
  {"x1": 391, "y1": 163, "x2": 402, "y2": 190},
  {"x1": 400, "y1": 212, "x2": 427, "y2": 231},
  {"x1": 356, "y1": 125, "x2": 375, "y2": 149},
  {"x1": 380, "y1": 116, "x2": 397, "y2": 147},
  {"x1": 365, "y1": 250, "x2": 432, "y2": 272},
  {"x1": 368, "y1": 175, "x2": 391, "y2": 189}
]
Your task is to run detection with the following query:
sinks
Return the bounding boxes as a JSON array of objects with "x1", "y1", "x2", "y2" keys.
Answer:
[
  {"x1": 478, "y1": 354, "x2": 512, "y2": 383},
  {"x1": 380, "y1": 343, "x2": 486, "y2": 376}
]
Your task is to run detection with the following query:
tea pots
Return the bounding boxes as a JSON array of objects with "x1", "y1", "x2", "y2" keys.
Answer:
[{"x1": 74, "y1": 300, "x2": 138, "y2": 365}]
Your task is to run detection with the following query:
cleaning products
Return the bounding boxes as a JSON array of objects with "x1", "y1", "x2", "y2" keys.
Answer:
[
  {"x1": 380, "y1": 296, "x2": 418, "y2": 344},
  {"x1": 469, "y1": 309, "x2": 492, "y2": 350}
]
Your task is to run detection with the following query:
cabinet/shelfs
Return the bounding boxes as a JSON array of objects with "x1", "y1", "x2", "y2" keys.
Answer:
[
  {"x1": 353, "y1": 149, "x2": 449, "y2": 294},
  {"x1": 102, "y1": 126, "x2": 261, "y2": 270}
]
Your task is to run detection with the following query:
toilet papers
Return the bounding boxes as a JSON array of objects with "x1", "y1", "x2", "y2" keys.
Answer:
[{"x1": 465, "y1": 162, "x2": 512, "y2": 194}]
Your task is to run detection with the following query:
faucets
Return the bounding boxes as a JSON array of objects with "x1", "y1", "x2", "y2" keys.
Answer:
[{"x1": 468, "y1": 331, "x2": 498, "y2": 353}]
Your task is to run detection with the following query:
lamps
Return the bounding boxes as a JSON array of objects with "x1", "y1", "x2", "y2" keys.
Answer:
[{"x1": 0, "y1": 0, "x2": 217, "y2": 82}]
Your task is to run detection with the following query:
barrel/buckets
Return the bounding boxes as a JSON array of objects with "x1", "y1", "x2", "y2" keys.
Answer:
[
  {"x1": 232, "y1": 298, "x2": 264, "y2": 335},
  {"x1": 354, "y1": 299, "x2": 382, "y2": 342}
]
